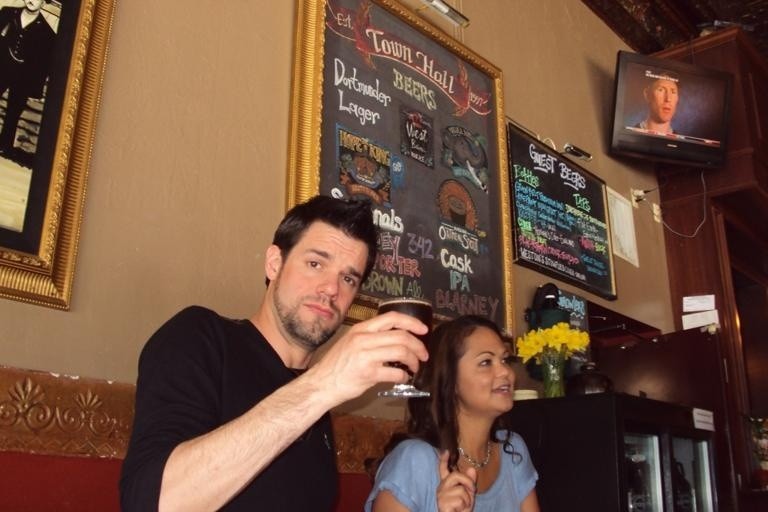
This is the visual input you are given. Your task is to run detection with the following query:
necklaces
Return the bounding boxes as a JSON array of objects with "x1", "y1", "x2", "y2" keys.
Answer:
[{"x1": 457, "y1": 441, "x2": 491, "y2": 468}]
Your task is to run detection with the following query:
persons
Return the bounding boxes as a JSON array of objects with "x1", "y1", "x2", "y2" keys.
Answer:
[
  {"x1": 363, "y1": 314, "x2": 542, "y2": 512},
  {"x1": 119, "y1": 195, "x2": 430, "y2": 512},
  {"x1": 632, "y1": 67, "x2": 681, "y2": 133}
]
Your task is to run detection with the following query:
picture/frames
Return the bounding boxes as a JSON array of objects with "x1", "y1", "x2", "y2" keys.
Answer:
[{"x1": 0, "y1": 0, "x2": 116, "y2": 315}]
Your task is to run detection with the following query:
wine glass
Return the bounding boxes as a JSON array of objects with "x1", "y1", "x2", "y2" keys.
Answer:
[{"x1": 375, "y1": 296, "x2": 433, "y2": 398}]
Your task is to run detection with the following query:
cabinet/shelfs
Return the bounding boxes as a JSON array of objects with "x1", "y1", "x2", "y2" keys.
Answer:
[{"x1": 504, "y1": 324, "x2": 758, "y2": 512}]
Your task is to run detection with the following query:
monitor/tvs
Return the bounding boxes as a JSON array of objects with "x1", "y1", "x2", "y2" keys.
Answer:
[{"x1": 607, "y1": 49, "x2": 735, "y2": 171}]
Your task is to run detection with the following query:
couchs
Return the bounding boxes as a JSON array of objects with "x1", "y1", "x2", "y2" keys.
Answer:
[{"x1": 1, "y1": 368, "x2": 400, "y2": 512}]
